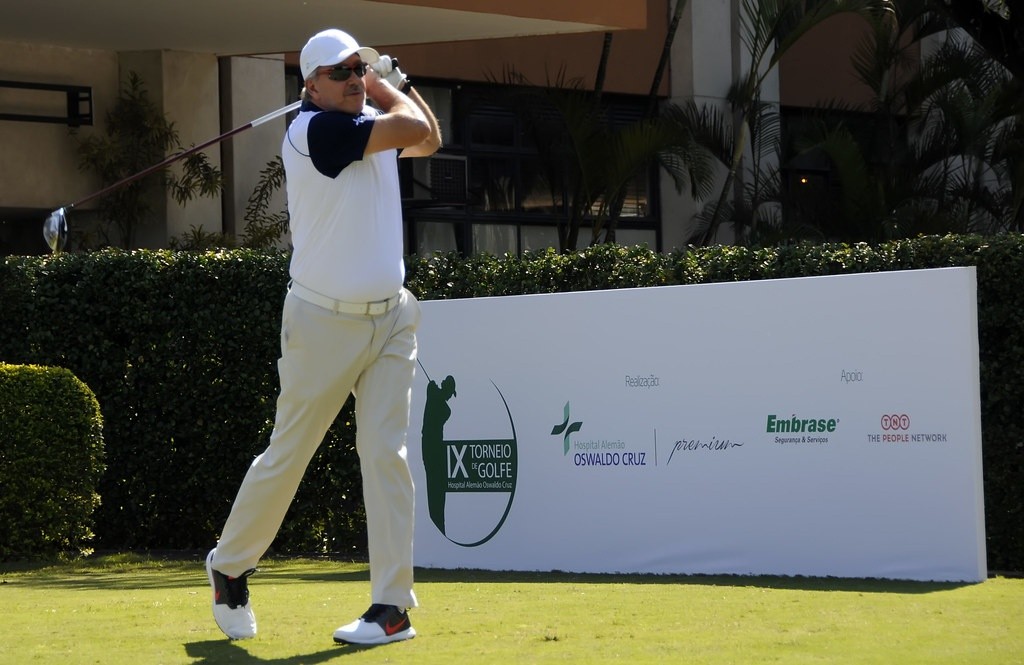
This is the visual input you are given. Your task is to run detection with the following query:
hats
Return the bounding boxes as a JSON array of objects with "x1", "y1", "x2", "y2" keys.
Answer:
[{"x1": 299, "y1": 27, "x2": 379, "y2": 80}]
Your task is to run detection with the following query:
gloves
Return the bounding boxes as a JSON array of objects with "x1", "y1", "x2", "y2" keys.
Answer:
[{"x1": 375, "y1": 54, "x2": 408, "y2": 90}]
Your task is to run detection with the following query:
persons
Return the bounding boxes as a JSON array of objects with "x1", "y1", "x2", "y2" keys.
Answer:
[{"x1": 206, "y1": 29, "x2": 442, "y2": 646}]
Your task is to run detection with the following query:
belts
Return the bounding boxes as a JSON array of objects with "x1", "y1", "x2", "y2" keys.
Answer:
[{"x1": 291, "y1": 282, "x2": 404, "y2": 317}]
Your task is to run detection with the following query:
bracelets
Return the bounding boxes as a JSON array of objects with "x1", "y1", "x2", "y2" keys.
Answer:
[{"x1": 401, "y1": 79, "x2": 411, "y2": 95}]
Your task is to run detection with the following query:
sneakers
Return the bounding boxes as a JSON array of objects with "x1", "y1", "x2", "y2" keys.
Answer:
[
  {"x1": 334, "y1": 602, "x2": 415, "y2": 645},
  {"x1": 206, "y1": 547, "x2": 257, "y2": 639}
]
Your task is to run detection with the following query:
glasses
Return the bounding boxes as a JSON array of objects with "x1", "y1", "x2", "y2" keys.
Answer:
[{"x1": 316, "y1": 60, "x2": 368, "y2": 81}]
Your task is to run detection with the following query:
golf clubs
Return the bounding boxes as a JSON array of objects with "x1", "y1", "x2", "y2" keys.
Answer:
[{"x1": 41, "y1": 57, "x2": 400, "y2": 255}]
